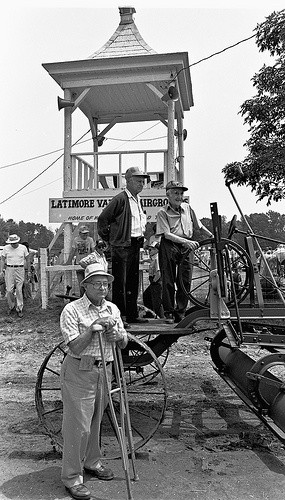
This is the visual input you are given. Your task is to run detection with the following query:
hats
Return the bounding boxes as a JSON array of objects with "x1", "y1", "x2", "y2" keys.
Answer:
[
  {"x1": 80, "y1": 263, "x2": 114, "y2": 286},
  {"x1": 166, "y1": 181, "x2": 188, "y2": 191},
  {"x1": 125, "y1": 167, "x2": 148, "y2": 178},
  {"x1": 22, "y1": 242, "x2": 29, "y2": 247},
  {"x1": 96, "y1": 240, "x2": 106, "y2": 247},
  {"x1": 79, "y1": 226, "x2": 90, "y2": 234},
  {"x1": 5, "y1": 235, "x2": 20, "y2": 243},
  {"x1": 146, "y1": 241, "x2": 160, "y2": 251}
]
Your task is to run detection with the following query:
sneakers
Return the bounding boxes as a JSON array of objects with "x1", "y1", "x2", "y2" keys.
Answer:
[
  {"x1": 84, "y1": 464, "x2": 114, "y2": 480},
  {"x1": 65, "y1": 484, "x2": 91, "y2": 500}
]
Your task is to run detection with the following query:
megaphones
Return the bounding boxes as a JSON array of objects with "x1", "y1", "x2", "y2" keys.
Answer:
[
  {"x1": 161, "y1": 86, "x2": 179, "y2": 101},
  {"x1": 175, "y1": 129, "x2": 187, "y2": 141},
  {"x1": 58, "y1": 96, "x2": 74, "y2": 111}
]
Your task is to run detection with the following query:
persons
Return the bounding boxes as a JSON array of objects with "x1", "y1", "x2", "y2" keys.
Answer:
[
  {"x1": 50, "y1": 251, "x2": 76, "y2": 266},
  {"x1": 22, "y1": 242, "x2": 38, "y2": 292},
  {"x1": 156, "y1": 181, "x2": 214, "y2": 323},
  {"x1": 80, "y1": 239, "x2": 112, "y2": 301},
  {"x1": 141, "y1": 242, "x2": 165, "y2": 318},
  {"x1": 97, "y1": 167, "x2": 149, "y2": 329},
  {"x1": 60, "y1": 264, "x2": 127, "y2": 500},
  {"x1": 62, "y1": 225, "x2": 96, "y2": 299},
  {"x1": 0, "y1": 234, "x2": 31, "y2": 318},
  {"x1": 198, "y1": 244, "x2": 285, "y2": 278}
]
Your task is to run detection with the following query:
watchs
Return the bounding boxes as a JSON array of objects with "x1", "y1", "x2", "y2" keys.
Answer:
[{"x1": 118, "y1": 334, "x2": 124, "y2": 344}]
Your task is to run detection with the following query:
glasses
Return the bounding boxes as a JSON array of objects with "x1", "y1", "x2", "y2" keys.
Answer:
[
  {"x1": 98, "y1": 246, "x2": 104, "y2": 249},
  {"x1": 149, "y1": 249, "x2": 152, "y2": 251},
  {"x1": 91, "y1": 282, "x2": 111, "y2": 290}
]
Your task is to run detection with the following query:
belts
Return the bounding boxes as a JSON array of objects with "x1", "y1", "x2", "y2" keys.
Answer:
[
  {"x1": 94, "y1": 360, "x2": 110, "y2": 368},
  {"x1": 7, "y1": 265, "x2": 23, "y2": 267}
]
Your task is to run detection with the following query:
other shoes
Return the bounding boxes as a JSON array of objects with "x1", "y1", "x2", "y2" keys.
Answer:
[
  {"x1": 122, "y1": 321, "x2": 132, "y2": 328},
  {"x1": 175, "y1": 316, "x2": 185, "y2": 323},
  {"x1": 17, "y1": 312, "x2": 23, "y2": 318},
  {"x1": 10, "y1": 306, "x2": 16, "y2": 318},
  {"x1": 126, "y1": 319, "x2": 148, "y2": 323},
  {"x1": 165, "y1": 316, "x2": 175, "y2": 323}
]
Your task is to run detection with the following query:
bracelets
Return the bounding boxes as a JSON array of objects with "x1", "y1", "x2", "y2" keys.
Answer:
[
  {"x1": 28, "y1": 270, "x2": 31, "y2": 274},
  {"x1": 182, "y1": 239, "x2": 187, "y2": 246}
]
